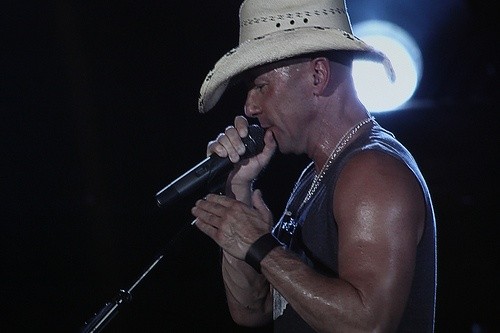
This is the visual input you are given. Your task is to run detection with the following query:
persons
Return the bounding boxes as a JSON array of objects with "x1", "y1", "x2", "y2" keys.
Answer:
[{"x1": 191, "y1": 0, "x2": 438, "y2": 333}]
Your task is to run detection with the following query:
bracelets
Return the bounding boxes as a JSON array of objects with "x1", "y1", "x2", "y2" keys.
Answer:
[{"x1": 244, "y1": 233, "x2": 287, "y2": 274}]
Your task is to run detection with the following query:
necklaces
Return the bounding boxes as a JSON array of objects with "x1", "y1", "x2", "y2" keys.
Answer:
[{"x1": 281, "y1": 116, "x2": 376, "y2": 234}]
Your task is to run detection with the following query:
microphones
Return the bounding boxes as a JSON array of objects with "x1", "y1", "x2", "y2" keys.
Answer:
[{"x1": 152, "y1": 123, "x2": 266, "y2": 206}]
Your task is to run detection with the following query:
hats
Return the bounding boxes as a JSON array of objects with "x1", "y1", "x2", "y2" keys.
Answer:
[{"x1": 198, "y1": 0, "x2": 396, "y2": 114}]
